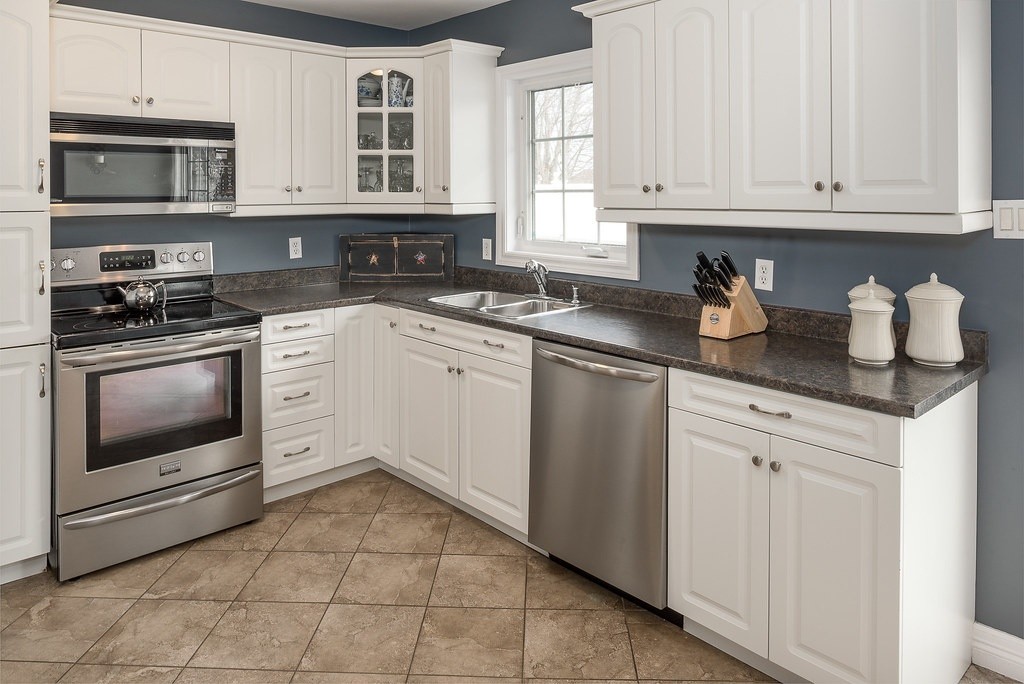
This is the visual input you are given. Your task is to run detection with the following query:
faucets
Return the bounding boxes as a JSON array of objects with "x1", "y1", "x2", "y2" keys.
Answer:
[
  {"x1": 524, "y1": 260, "x2": 552, "y2": 299},
  {"x1": 571, "y1": 285, "x2": 581, "y2": 304}
]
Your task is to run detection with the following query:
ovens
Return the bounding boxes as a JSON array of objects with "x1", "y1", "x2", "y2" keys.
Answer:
[{"x1": 45, "y1": 324, "x2": 265, "y2": 585}]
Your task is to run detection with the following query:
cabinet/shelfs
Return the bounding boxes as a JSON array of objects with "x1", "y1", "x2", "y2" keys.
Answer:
[
  {"x1": 728, "y1": 0, "x2": 992, "y2": 213},
  {"x1": 667, "y1": 366, "x2": 978, "y2": 684},
  {"x1": 346, "y1": 58, "x2": 424, "y2": 203},
  {"x1": 230, "y1": 43, "x2": 346, "y2": 205},
  {"x1": 374, "y1": 304, "x2": 399, "y2": 469},
  {"x1": 1, "y1": 0, "x2": 51, "y2": 568},
  {"x1": 335, "y1": 303, "x2": 373, "y2": 468},
  {"x1": 424, "y1": 51, "x2": 497, "y2": 203},
  {"x1": 399, "y1": 307, "x2": 533, "y2": 535},
  {"x1": 592, "y1": 0, "x2": 729, "y2": 209},
  {"x1": 261, "y1": 307, "x2": 335, "y2": 489},
  {"x1": 50, "y1": 17, "x2": 230, "y2": 122}
]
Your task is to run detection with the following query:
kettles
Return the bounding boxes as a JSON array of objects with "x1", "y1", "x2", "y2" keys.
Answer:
[{"x1": 116, "y1": 276, "x2": 167, "y2": 310}]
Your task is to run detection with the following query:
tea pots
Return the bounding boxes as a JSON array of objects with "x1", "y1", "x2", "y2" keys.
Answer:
[{"x1": 381, "y1": 74, "x2": 411, "y2": 107}]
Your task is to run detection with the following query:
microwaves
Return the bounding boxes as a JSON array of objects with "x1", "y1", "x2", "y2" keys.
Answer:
[{"x1": 49, "y1": 111, "x2": 237, "y2": 218}]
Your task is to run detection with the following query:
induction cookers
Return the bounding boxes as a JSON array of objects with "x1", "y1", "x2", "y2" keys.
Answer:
[{"x1": 50, "y1": 241, "x2": 263, "y2": 353}]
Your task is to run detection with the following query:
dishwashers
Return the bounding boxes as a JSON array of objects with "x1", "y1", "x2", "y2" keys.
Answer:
[{"x1": 527, "y1": 339, "x2": 669, "y2": 613}]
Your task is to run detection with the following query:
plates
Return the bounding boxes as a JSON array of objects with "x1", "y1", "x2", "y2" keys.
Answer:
[{"x1": 358, "y1": 98, "x2": 383, "y2": 107}]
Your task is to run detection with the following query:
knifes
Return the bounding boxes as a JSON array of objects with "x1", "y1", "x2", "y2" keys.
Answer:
[{"x1": 690, "y1": 249, "x2": 740, "y2": 309}]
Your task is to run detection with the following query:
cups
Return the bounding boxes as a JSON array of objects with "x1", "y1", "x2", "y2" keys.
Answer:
[
  {"x1": 358, "y1": 120, "x2": 413, "y2": 150},
  {"x1": 405, "y1": 96, "x2": 413, "y2": 107},
  {"x1": 375, "y1": 170, "x2": 413, "y2": 192}
]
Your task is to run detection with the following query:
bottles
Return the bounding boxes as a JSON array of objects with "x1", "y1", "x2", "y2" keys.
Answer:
[
  {"x1": 848, "y1": 290, "x2": 895, "y2": 365},
  {"x1": 847, "y1": 274, "x2": 897, "y2": 349},
  {"x1": 905, "y1": 272, "x2": 965, "y2": 367}
]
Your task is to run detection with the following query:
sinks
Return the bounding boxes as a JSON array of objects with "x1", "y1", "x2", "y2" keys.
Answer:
[
  {"x1": 477, "y1": 297, "x2": 594, "y2": 320},
  {"x1": 427, "y1": 291, "x2": 537, "y2": 311}
]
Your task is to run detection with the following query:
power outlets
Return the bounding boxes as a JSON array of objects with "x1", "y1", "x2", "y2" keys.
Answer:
[
  {"x1": 755, "y1": 258, "x2": 774, "y2": 291},
  {"x1": 483, "y1": 239, "x2": 491, "y2": 261},
  {"x1": 289, "y1": 237, "x2": 302, "y2": 259}
]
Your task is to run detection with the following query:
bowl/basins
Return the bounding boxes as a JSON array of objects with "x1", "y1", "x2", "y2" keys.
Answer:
[{"x1": 358, "y1": 76, "x2": 380, "y2": 97}]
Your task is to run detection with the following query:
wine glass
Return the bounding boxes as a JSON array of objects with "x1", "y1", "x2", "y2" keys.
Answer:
[{"x1": 358, "y1": 158, "x2": 407, "y2": 192}]
